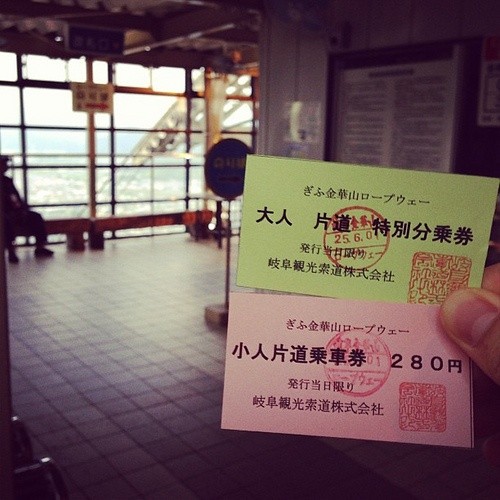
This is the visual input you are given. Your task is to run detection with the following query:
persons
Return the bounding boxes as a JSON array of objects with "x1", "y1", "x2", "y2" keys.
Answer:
[
  {"x1": 1, "y1": 154, "x2": 56, "y2": 262},
  {"x1": 442, "y1": 257, "x2": 499, "y2": 387}
]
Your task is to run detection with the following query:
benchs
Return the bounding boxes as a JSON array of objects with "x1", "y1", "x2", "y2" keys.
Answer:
[
  {"x1": 89, "y1": 207, "x2": 213, "y2": 252},
  {"x1": 44, "y1": 212, "x2": 91, "y2": 255}
]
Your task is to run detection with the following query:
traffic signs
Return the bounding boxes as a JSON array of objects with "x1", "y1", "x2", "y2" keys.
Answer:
[
  {"x1": 204, "y1": 138, "x2": 253, "y2": 200},
  {"x1": 72, "y1": 81, "x2": 113, "y2": 113}
]
